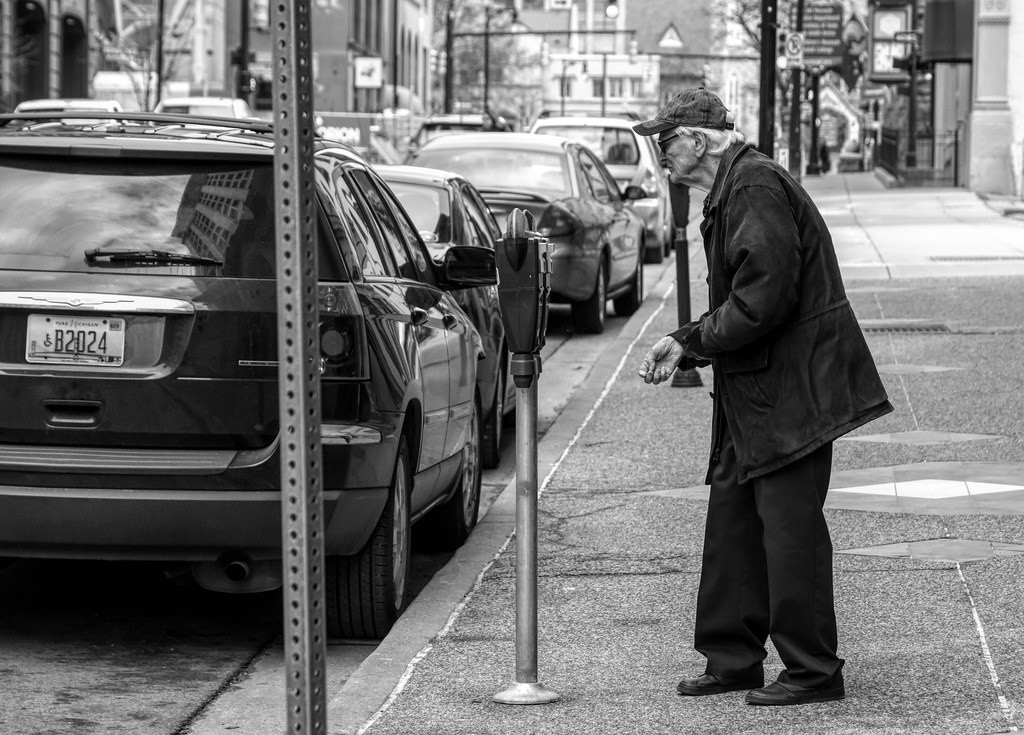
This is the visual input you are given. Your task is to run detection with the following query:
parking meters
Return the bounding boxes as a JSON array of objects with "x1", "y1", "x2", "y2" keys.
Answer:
[{"x1": 492, "y1": 204, "x2": 564, "y2": 706}]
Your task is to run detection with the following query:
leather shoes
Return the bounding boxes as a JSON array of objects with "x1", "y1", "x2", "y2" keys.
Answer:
[
  {"x1": 677, "y1": 675, "x2": 765, "y2": 696},
  {"x1": 746, "y1": 683, "x2": 845, "y2": 705}
]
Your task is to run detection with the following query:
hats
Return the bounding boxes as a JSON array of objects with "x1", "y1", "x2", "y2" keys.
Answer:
[{"x1": 632, "y1": 87, "x2": 735, "y2": 136}]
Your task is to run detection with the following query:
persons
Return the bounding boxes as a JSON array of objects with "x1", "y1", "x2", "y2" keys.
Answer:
[
  {"x1": 820, "y1": 138, "x2": 831, "y2": 173},
  {"x1": 631, "y1": 87, "x2": 898, "y2": 707}
]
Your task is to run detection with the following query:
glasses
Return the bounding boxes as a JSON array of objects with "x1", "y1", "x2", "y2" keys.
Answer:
[{"x1": 657, "y1": 132, "x2": 685, "y2": 152}]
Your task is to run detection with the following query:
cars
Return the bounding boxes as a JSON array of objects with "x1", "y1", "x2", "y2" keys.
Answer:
[
  {"x1": 408, "y1": 129, "x2": 648, "y2": 335},
  {"x1": 10, "y1": 97, "x2": 130, "y2": 127},
  {"x1": 399, "y1": 101, "x2": 675, "y2": 264},
  {"x1": 370, "y1": 160, "x2": 517, "y2": 472},
  {"x1": 148, "y1": 96, "x2": 255, "y2": 127}
]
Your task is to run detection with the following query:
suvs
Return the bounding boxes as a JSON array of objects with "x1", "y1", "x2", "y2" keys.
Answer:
[{"x1": 0, "y1": 108, "x2": 501, "y2": 646}]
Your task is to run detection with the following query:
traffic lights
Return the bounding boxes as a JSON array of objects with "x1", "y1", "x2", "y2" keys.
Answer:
[{"x1": 776, "y1": 27, "x2": 790, "y2": 72}]
